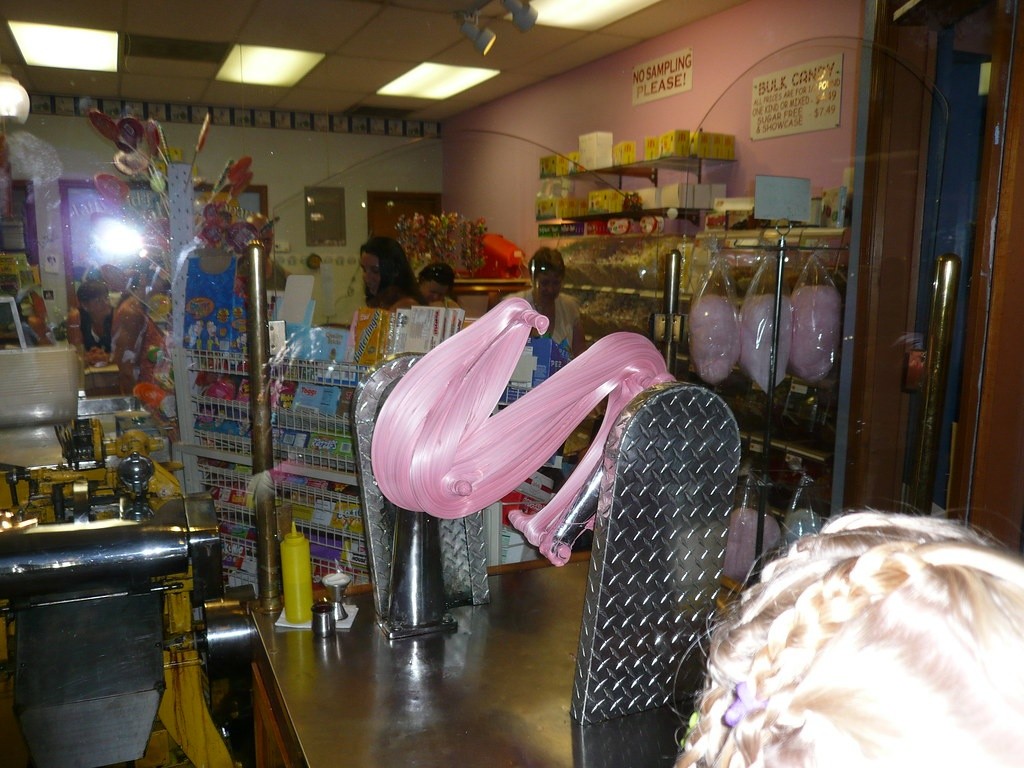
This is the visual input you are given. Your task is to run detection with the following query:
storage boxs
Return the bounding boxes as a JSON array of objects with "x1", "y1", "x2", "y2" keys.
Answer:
[
  {"x1": 537, "y1": 125, "x2": 855, "y2": 239},
  {"x1": 182, "y1": 257, "x2": 564, "y2": 595}
]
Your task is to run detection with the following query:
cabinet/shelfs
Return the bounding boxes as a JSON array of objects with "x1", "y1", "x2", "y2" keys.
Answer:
[
  {"x1": 537, "y1": 158, "x2": 851, "y2": 591},
  {"x1": 171, "y1": 342, "x2": 494, "y2": 612}
]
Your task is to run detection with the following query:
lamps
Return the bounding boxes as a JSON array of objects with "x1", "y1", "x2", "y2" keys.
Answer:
[
  {"x1": 500, "y1": 0, "x2": 539, "y2": 32},
  {"x1": 1, "y1": 64, "x2": 31, "y2": 123},
  {"x1": 461, "y1": 20, "x2": 498, "y2": 57}
]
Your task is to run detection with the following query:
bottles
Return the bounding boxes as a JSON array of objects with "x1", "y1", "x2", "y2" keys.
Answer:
[{"x1": 280, "y1": 522, "x2": 313, "y2": 624}]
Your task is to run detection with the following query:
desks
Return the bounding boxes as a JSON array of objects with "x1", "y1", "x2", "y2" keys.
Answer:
[{"x1": 248, "y1": 551, "x2": 751, "y2": 767}]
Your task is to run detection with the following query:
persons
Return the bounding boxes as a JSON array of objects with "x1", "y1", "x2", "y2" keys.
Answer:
[
  {"x1": 0, "y1": 284, "x2": 41, "y2": 348},
  {"x1": 501, "y1": 247, "x2": 587, "y2": 457},
  {"x1": 357, "y1": 236, "x2": 462, "y2": 314},
  {"x1": 76, "y1": 254, "x2": 173, "y2": 397},
  {"x1": 673, "y1": 506, "x2": 1024, "y2": 768}
]
